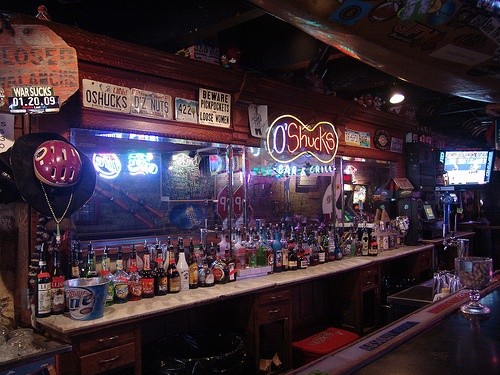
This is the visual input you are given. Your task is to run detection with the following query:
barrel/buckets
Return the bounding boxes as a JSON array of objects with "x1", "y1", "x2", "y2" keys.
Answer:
[{"x1": 63, "y1": 277, "x2": 112, "y2": 321}]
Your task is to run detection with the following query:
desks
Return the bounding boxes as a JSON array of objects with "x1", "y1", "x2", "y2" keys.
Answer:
[{"x1": 281, "y1": 268, "x2": 500, "y2": 375}]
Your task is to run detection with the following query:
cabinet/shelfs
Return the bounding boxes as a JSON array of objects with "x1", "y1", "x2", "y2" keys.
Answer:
[
  {"x1": 405, "y1": 142, "x2": 437, "y2": 191},
  {"x1": 60, "y1": 320, "x2": 143, "y2": 375},
  {"x1": 229, "y1": 248, "x2": 437, "y2": 375}
]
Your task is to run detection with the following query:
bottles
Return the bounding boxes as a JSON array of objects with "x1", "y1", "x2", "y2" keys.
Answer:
[
  {"x1": 113, "y1": 245, "x2": 128, "y2": 304},
  {"x1": 140, "y1": 244, "x2": 154, "y2": 298},
  {"x1": 176, "y1": 248, "x2": 189, "y2": 291},
  {"x1": 186, "y1": 199, "x2": 406, "y2": 289},
  {"x1": 167, "y1": 245, "x2": 181, "y2": 294},
  {"x1": 67, "y1": 241, "x2": 87, "y2": 279},
  {"x1": 127, "y1": 244, "x2": 143, "y2": 301},
  {"x1": 154, "y1": 248, "x2": 167, "y2": 296},
  {"x1": 175, "y1": 237, "x2": 185, "y2": 264},
  {"x1": 34, "y1": 242, "x2": 52, "y2": 318},
  {"x1": 50, "y1": 251, "x2": 65, "y2": 315},
  {"x1": 433, "y1": 288, "x2": 450, "y2": 302},
  {"x1": 85, "y1": 243, "x2": 98, "y2": 278},
  {"x1": 99, "y1": 245, "x2": 115, "y2": 307},
  {"x1": 163, "y1": 236, "x2": 174, "y2": 271}
]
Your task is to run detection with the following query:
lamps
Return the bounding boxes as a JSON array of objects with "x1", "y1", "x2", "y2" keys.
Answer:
[{"x1": 388, "y1": 81, "x2": 405, "y2": 104}]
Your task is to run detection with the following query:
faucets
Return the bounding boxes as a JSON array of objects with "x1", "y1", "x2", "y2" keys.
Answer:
[
  {"x1": 201, "y1": 224, "x2": 218, "y2": 250},
  {"x1": 443, "y1": 231, "x2": 469, "y2": 257}
]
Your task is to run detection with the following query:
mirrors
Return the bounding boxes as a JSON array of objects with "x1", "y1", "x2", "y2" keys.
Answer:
[{"x1": 69, "y1": 127, "x2": 399, "y2": 251}]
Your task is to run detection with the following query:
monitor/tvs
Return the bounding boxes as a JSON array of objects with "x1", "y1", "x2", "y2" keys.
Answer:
[{"x1": 439, "y1": 150, "x2": 494, "y2": 185}]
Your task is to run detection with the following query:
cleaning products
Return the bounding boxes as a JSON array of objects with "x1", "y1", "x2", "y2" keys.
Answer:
[{"x1": 433, "y1": 288, "x2": 449, "y2": 302}]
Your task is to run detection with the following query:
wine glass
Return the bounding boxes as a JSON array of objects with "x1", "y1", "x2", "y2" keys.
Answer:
[{"x1": 454, "y1": 257, "x2": 493, "y2": 315}]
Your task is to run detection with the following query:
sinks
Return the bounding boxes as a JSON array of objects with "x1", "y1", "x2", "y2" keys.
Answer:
[
  {"x1": 0, "y1": 333, "x2": 72, "y2": 375},
  {"x1": 428, "y1": 269, "x2": 497, "y2": 285},
  {"x1": 387, "y1": 285, "x2": 433, "y2": 303}
]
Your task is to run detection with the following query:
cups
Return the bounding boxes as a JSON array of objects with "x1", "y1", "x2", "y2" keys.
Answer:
[
  {"x1": 432, "y1": 270, "x2": 460, "y2": 293},
  {"x1": 0, "y1": 325, "x2": 39, "y2": 361}
]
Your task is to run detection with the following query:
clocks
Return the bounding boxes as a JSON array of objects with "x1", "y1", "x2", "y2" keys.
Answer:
[{"x1": 373, "y1": 128, "x2": 391, "y2": 150}]
